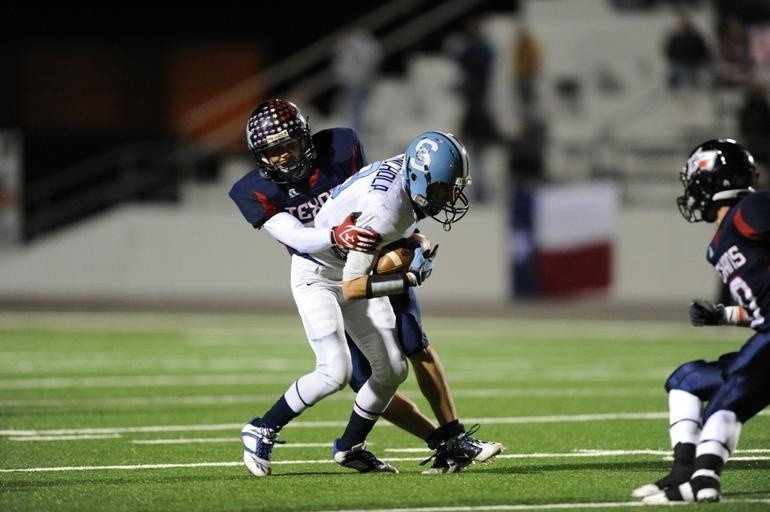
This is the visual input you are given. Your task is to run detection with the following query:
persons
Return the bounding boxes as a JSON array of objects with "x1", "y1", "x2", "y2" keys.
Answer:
[
  {"x1": 659, "y1": 6, "x2": 721, "y2": 92},
  {"x1": 452, "y1": 14, "x2": 612, "y2": 296},
  {"x1": 631, "y1": 137, "x2": 770, "y2": 506},
  {"x1": 333, "y1": 17, "x2": 383, "y2": 128},
  {"x1": 228, "y1": 98, "x2": 505, "y2": 476},
  {"x1": 239, "y1": 129, "x2": 474, "y2": 477}
]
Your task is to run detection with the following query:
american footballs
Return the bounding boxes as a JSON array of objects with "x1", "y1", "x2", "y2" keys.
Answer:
[{"x1": 376, "y1": 247, "x2": 414, "y2": 277}]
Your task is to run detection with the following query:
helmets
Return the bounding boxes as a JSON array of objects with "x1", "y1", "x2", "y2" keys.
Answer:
[
  {"x1": 676, "y1": 138, "x2": 757, "y2": 223},
  {"x1": 240, "y1": 100, "x2": 315, "y2": 185},
  {"x1": 403, "y1": 131, "x2": 470, "y2": 231}
]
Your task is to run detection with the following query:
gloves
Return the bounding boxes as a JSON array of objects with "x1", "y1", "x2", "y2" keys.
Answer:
[
  {"x1": 332, "y1": 212, "x2": 381, "y2": 252},
  {"x1": 408, "y1": 243, "x2": 439, "y2": 288},
  {"x1": 689, "y1": 301, "x2": 726, "y2": 327}
]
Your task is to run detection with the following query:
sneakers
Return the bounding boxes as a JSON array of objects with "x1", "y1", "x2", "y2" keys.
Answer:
[
  {"x1": 419, "y1": 433, "x2": 502, "y2": 475},
  {"x1": 333, "y1": 439, "x2": 398, "y2": 473},
  {"x1": 631, "y1": 467, "x2": 720, "y2": 505},
  {"x1": 240, "y1": 418, "x2": 278, "y2": 477}
]
